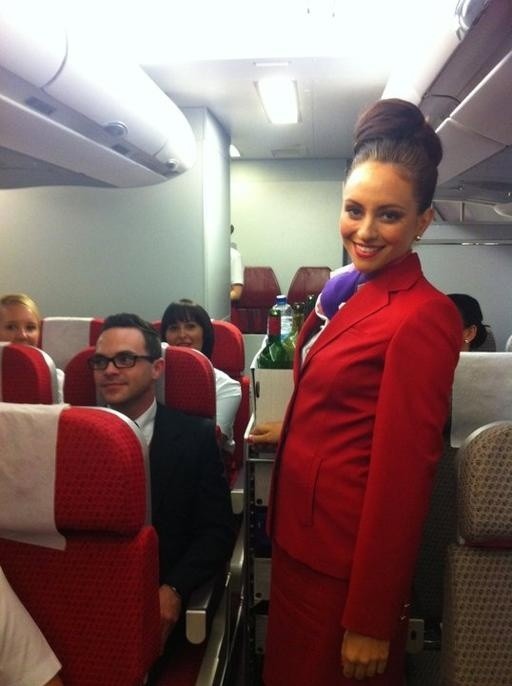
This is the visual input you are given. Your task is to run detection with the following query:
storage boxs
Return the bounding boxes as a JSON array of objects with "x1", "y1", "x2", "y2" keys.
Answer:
[
  {"x1": 254, "y1": 453, "x2": 275, "y2": 506},
  {"x1": 251, "y1": 334, "x2": 295, "y2": 422},
  {"x1": 255, "y1": 615, "x2": 267, "y2": 654},
  {"x1": 254, "y1": 558, "x2": 271, "y2": 600}
]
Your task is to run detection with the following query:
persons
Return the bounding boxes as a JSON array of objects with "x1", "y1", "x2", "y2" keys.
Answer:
[
  {"x1": 1, "y1": 293, "x2": 66, "y2": 405},
  {"x1": 87, "y1": 313, "x2": 232, "y2": 672},
  {"x1": 160, "y1": 301, "x2": 243, "y2": 450},
  {"x1": 229, "y1": 225, "x2": 246, "y2": 304},
  {"x1": 1, "y1": 560, "x2": 67, "y2": 686},
  {"x1": 244, "y1": 98, "x2": 463, "y2": 686},
  {"x1": 444, "y1": 294, "x2": 488, "y2": 354}
]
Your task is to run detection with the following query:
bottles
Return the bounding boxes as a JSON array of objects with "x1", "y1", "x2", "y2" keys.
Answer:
[{"x1": 268, "y1": 296, "x2": 315, "y2": 366}]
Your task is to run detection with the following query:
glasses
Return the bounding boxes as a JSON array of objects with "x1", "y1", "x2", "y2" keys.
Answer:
[{"x1": 87, "y1": 351, "x2": 154, "y2": 369}]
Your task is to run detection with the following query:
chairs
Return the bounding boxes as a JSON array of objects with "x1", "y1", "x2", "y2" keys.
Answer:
[
  {"x1": 149, "y1": 319, "x2": 250, "y2": 602},
  {"x1": 239, "y1": 266, "x2": 281, "y2": 334},
  {"x1": 64, "y1": 346, "x2": 231, "y2": 686},
  {"x1": 0, "y1": 402, "x2": 162, "y2": 686},
  {"x1": 450, "y1": 351, "x2": 512, "y2": 448},
  {"x1": 441, "y1": 419, "x2": 512, "y2": 685},
  {"x1": 287, "y1": 266, "x2": 332, "y2": 315},
  {"x1": 37, "y1": 317, "x2": 105, "y2": 371},
  {"x1": 0, "y1": 341, "x2": 58, "y2": 405}
]
